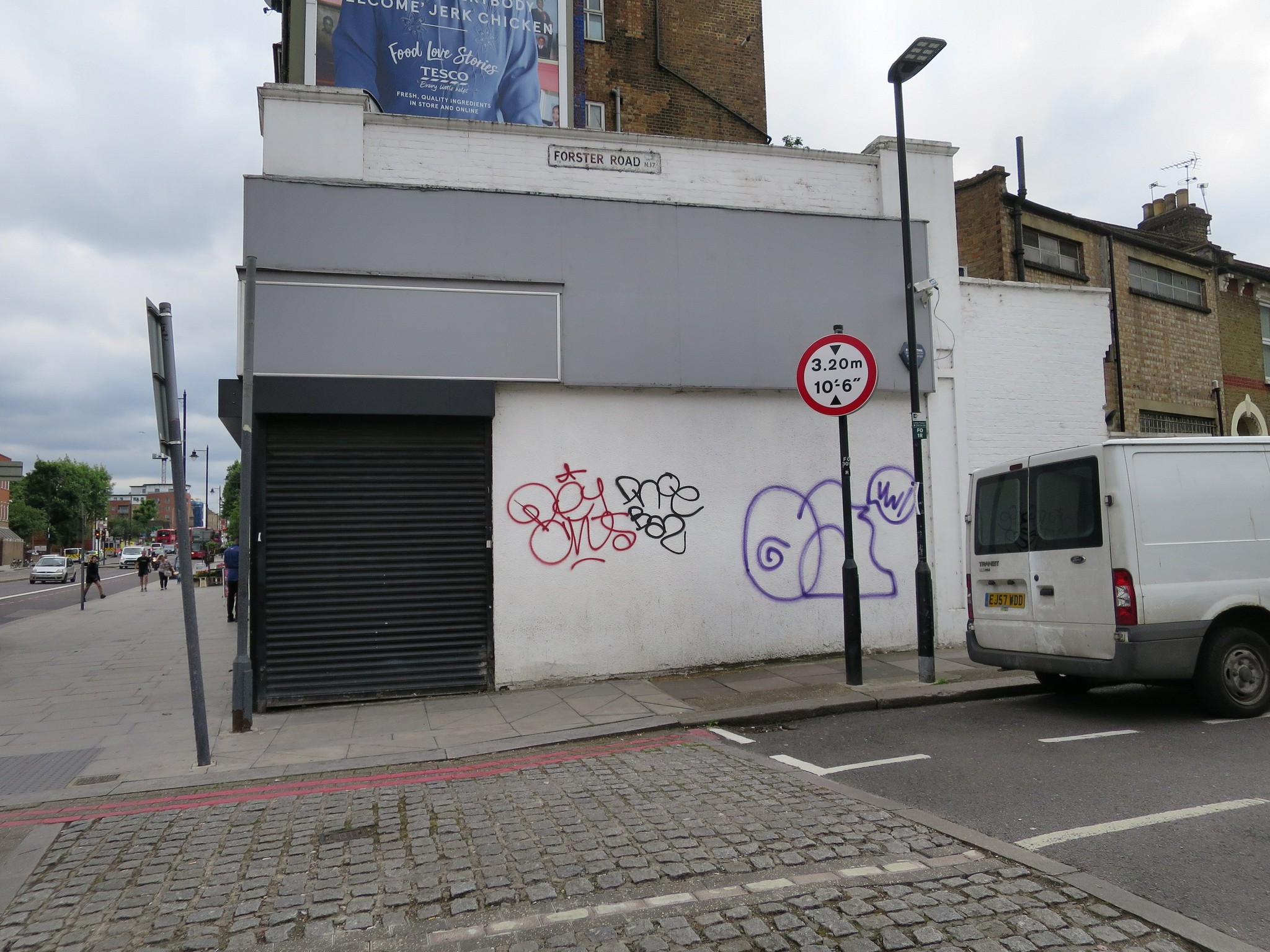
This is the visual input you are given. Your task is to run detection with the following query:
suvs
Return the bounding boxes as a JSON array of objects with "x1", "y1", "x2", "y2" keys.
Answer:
[
  {"x1": 151, "y1": 543, "x2": 164, "y2": 551},
  {"x1": 119, "y1": 546, "x2": 149, "y2": 569}
]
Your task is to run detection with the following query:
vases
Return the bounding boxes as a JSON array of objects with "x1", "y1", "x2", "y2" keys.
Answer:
[
  {"x1": 199, "y1": 577, "x2": 209, "y2": 588},
  {"x1": 210, "y1": 576, "x2": 222, "y2": 586}
]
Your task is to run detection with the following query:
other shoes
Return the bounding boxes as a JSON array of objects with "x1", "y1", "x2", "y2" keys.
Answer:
[
  {"x1": 161, "y1": 588, "x2": 163, "y2": 590},
  {"x1": 227, "y1": 618, "x2": 233, "y2": 622},
  {"x1": 165, "y1": 588, "x2": 167, "y2": 589},
  {"x1": 84, "y1": 598, "x2": 86, "y2": 602},
  {"x1": 177, "y1": 582, "x2": 180, "y2": 584},
  {"x1": 144, "y1": 588, "x2": 147, "y2": 591},
  {"x1": 100, "y1": 595, "x2": 106, "y2": 598},
  {"x1": 235, "y1": 618, "x2": 238, "y2": 623},
  {"x1": 141, "y1": 588, "x2": 143, "y2": 591}
]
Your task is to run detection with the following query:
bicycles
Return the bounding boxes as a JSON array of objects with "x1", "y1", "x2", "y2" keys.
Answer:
[{"x1": 10, "y1": 559, "x2": 29, "y2": 569}]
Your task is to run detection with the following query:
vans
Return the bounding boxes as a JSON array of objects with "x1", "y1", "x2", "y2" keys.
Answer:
[{"x1": 964, "y1": 435, "x2": 1269, "y2": 719}]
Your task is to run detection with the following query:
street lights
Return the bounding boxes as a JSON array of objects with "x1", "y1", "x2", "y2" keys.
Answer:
[
  {"x1": 188, "y1": 445, "x2": 209, "y2": 527},
  {"x1": 887, "y1": 35, "x2": 946, "y2": 693},
  {"x1": 210, "y1": 485, "x2": 220, "y2": 530}
]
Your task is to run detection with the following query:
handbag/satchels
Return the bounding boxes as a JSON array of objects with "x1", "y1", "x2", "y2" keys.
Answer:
[{"x1": 164, "y1": 571, "x2": 172, "y2": 577}]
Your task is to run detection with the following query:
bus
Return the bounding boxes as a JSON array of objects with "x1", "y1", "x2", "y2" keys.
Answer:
[
  {"x1": 104, "y1": 540, "x2": 120, "y2": 556},
  {"x1": 189, "y1": 527, "x2": 216, "y2": 562},
  {"x1": 156, "y1": 529, "x2": 175, "y2": 554},
  {"x1": 64, "y1": 548, "x2": 82, "y2": 563},
  {"x1": 212, "y1": 529, "x2": 221, "y2": 551}
]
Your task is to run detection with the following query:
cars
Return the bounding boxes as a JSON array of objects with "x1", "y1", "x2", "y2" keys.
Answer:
[
  {"x1": 29, "y1": 556, "x2": 76, "y2": 584},
  {"x1": 37, "y1": 554, "x2": 62, "y2": 564},
  {"x1": 28, "y1": 550, "x2": 40, "y2": 556},
  {"x1": 163, "y1": 544, "x2": 177, "y2": 555},
  {"x1": 84, "y1": 550, "x2": 107, "y2": 565}
]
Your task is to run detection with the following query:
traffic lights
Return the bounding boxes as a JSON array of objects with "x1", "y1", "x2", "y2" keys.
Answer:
[
  {"x1": 101, "y1": 530, "x2": 106, "y2": 540},
  {"x1": 95, "y1": 529, "x2": 100, "y2": 539}
]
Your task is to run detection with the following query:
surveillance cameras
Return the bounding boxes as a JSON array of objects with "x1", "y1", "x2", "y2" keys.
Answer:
[{"x1": 913, "y1": 278, "x2": 939, "y2": 294}]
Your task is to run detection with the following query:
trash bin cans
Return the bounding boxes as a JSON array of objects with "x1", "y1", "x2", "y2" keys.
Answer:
[{"x1": 208, "y1": 554, "x2": 216, "y2": 563}]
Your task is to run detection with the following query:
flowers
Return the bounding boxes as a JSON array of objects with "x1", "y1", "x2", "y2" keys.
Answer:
[{"x1": 195, "y1": 571, "x2": 210, "y2": 578}]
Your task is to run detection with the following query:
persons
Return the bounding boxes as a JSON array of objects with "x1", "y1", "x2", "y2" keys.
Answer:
[
  {"x1": 224, "y1": 535, "x2": 239, "y2": 622},
  {"x1": 531, "y1": 0, "x2": 553, "y2": 32},
  {"x1": 84, "y1": 556, "x2": 106, "y2": 602},
  {"x1": 536, "y1": 37, "x2": 548, "y2": 57},
  {"x1": 547, "y1": 105, "x2": 560, "y2": 127},
  {"x1": 332, "y1": 0, "x2": 544, "y2": 126},
  {"x1": 134, "y1": 550, "x2": 151, "y2": 592},
  {"x1": 317, "y1": 15, "x2": 335, "y2": 60},
  {"x1": 174, "y1": 551, "x2": 181, "y2": 584},
  {"x1": 156, "y1": 554, "x2": 176, "y2": 591}
]
